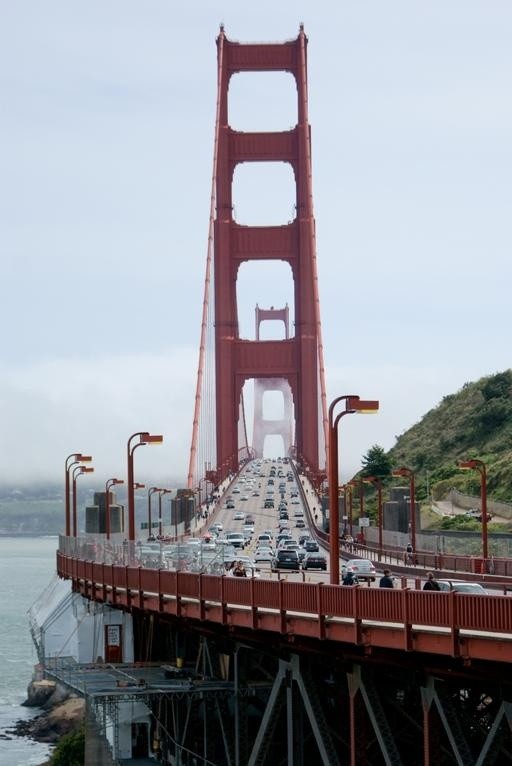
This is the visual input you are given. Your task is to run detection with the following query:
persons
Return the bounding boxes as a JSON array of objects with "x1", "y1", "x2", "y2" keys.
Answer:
[
  {"x1": 379, "y1": 569, "x2": 393, "y2": 589},
  {"x1": 422, "y1": 573, "x2": 440, "y2": 591},
  {"x1": 115, "y1": 534, "x2": 248, "y2": 578},
  {"x1": 342, "y1": 567, "x2": 358, "y2": 585},
  {"x1": 405, "y1": 543, "x2": 412, "y2": 562}
]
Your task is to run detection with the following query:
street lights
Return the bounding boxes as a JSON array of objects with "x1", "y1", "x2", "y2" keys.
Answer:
[
  {"x1": 63, "y1": 452, "x2": 93, "y2": 535},
  {"x1": 337, "y1": 478, "x2": 364, "y2": 542},
  {"x1": 459, "y1": 459, "x2": 490, "y2": 574},
  {"x1": 105, "y1": 477, "x2": 124, "y2": 539},
  {"x1": 126, "y1": 432, "x2": 164, "y2": 543},
  {"x1": 327, "y1": 394, "x2": 380, "y2": 591},
  {"x1": 362, "y1": 475, "x2": 384, "y2": 550},
  {"x1": 393, "y1": 465, "x2": 417, "y2": 568},
  {"x1": 72, "y1": 464, "x2": 93, "y2": 538}
]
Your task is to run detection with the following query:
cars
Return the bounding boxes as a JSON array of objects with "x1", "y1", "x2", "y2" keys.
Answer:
[
  {"x1": 436, "y1": 577, "x2": 491, "y2": 595},
  {"x1": 341, "y1": 558, "x2": 376, "y2": 581},
  {"x1": 133, "y1": 456, "x2": 328, "y2": 579}
]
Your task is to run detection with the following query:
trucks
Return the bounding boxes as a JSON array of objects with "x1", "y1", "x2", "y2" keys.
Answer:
[{"x1": 462, "y1": 507, "x2": 493, "y2": 521}]
